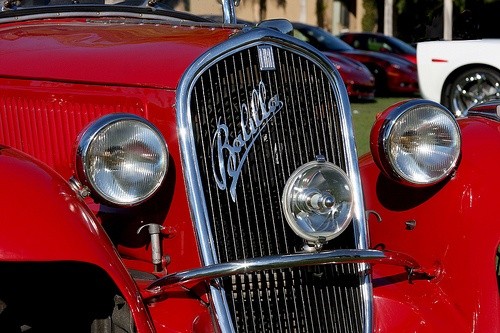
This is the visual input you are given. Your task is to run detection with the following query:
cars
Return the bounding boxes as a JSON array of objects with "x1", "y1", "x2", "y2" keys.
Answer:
[{"x1": 1, "y1": 0, "x2": 500, "y2": 333}]
